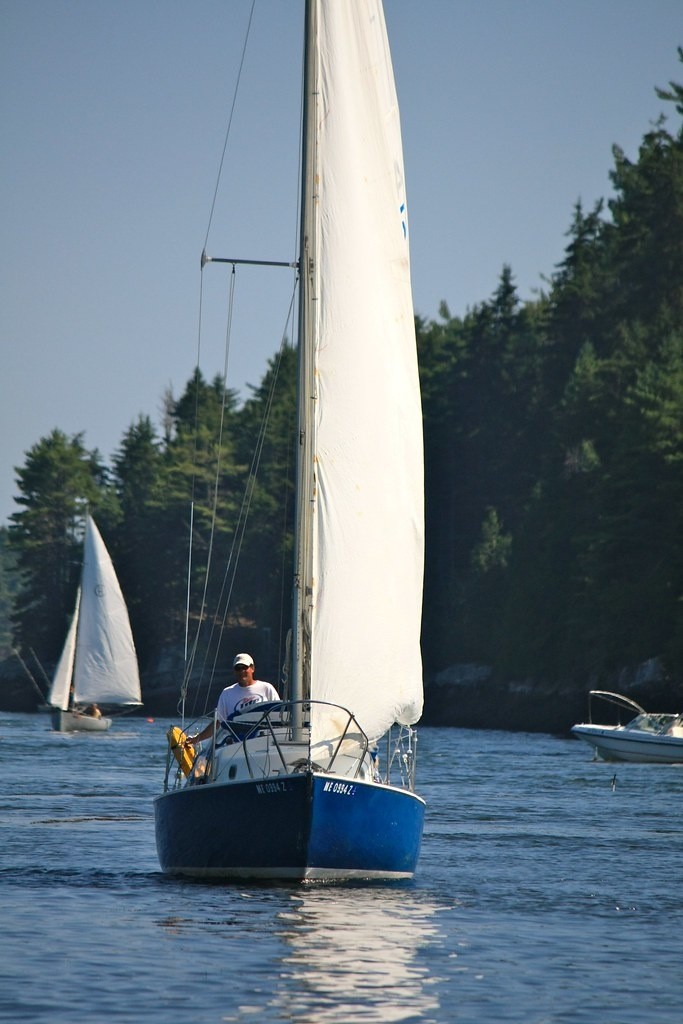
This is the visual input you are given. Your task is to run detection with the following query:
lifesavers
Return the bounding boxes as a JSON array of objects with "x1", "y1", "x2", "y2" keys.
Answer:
[{"x1": 166, "y1": 726, "x2": 197, "y2": 776}]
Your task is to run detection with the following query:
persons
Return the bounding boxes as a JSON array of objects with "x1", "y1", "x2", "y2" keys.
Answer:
[
  {"x1": 83, "y1": 704, "x2": 101, "y2": 719},
  {"x1": 69, "y1": 686, "x2": 74, "y2": 707},
  {"x1": 186, "y1": 653, "x2": 280, "y2": 744}
]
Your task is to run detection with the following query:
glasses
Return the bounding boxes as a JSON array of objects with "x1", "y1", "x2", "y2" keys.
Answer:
[{"x1": 235, "y1": 664, "x2": 252, "y2": 671}]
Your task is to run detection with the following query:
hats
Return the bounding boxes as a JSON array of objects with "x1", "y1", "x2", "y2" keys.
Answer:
[{"x1": 233, "y1": 653, "x2": 254, "y2": 666}]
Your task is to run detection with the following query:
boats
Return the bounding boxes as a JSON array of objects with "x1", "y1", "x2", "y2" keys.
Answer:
[{"x1": 571, "y1": 690, "x2": 683, "y2": 763}]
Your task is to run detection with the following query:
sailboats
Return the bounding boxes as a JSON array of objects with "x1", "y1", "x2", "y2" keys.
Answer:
[
  {"x1": 152, "y1": 1, "x2": 426, "y2": 887},
  {"x1": 47, "y1": 514, "x2": 144, "y2": 732}
]
